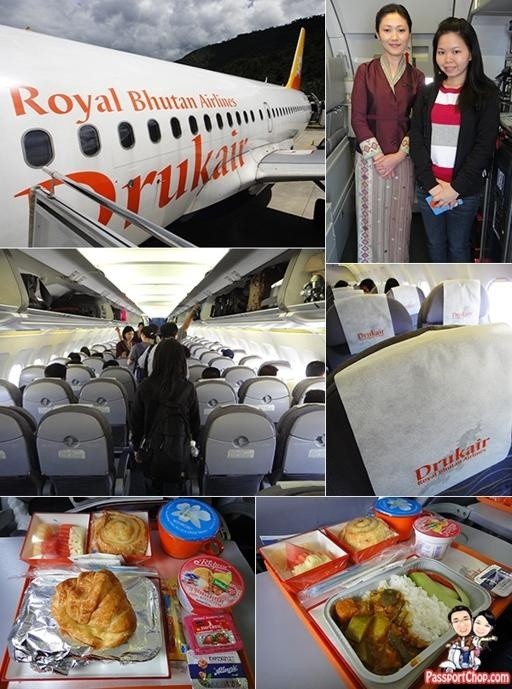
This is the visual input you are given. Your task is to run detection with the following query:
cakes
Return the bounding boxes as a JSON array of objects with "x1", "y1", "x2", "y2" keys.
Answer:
[
  {"x1": 337, "y1": 516, "x2": 395, "y2": 551},
  {"x1": 58, "y1": 523, "x2": 85, "y2": 556},
  {"x1": 32, "y1": 523, "x2": 58, "y2": 559},
  {"x1": 286, "y1": 545, "x2": 336, "y2": 577}
]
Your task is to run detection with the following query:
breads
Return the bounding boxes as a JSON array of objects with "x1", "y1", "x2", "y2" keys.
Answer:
[
  {"x1": 53, "y1": 568, "x2": 137, "y2": 650},
  {"x1": 91, "y1": 511, "x2": 149, "y2": 558}
]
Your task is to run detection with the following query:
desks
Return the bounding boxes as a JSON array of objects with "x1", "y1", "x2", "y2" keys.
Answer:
[
  {"x1": 467, "y1": 501, "x2": 511, "y2": 541},
  {"x1": 256, "y1": 520, "x2": 511, "y2": 689},
  {"x1": 0, "y1": 536, "x2": 254, "y2": 688}
]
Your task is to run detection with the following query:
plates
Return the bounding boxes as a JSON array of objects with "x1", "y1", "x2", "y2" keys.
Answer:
[
  {"x1": 321, "y1": 556, "x2": 494, "y2": 682},
  {"x1": 2, "y1": 570, "x2": 173, "y2": 682}
]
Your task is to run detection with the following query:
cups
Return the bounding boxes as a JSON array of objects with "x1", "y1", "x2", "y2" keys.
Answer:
[
  {"x1": 373, "y1": 498, "x2": 461, "y2": 558},
  {"x1": 156, "y1": 497, "x2": 224, "y2": 560},
  {"x1": 176, "y1": 555, "x2": 246, "y2": 615}
]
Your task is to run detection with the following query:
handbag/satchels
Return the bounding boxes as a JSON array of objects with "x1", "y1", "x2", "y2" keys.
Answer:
[
  {"x1": 133, "y1": 379, "x2": 195, "y2": 486},
  {"x1": 192, "y1": 260, "x2": 290, "y2": 322},
  {"x1": 19, "y1": 272, "x2": 127, "y2": 323}
]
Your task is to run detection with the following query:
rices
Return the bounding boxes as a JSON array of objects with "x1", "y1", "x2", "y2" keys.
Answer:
[{"x1": 360, "y1": 574, "x2": 454, "y2": 644}]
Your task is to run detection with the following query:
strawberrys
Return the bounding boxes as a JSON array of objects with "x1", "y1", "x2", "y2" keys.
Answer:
[{"x1": 203, "y1": 633, "x2": 231, "y2": 645}]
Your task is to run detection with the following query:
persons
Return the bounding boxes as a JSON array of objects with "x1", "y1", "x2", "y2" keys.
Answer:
[
  {"x1": 384, "y1": 278, "x2": 400, "y2": 294},
  {"x1": 258, "y1": 364, "x2": 278, "y2": 376},
  {"x1": 471, "y1": 610, "x2": 498, "y2": 667},
  {"x1": 409, "y1": 16, "x2": 500, "y2": 263},
  {"x1": 223, "y1": 349, "x2": 235, "y2": 357},
  {"x1": 350, "y1": 4, "x2": 424, "y2": 263},
  {"x1": 358, "y1": 279, "x2": 378, "y2": 293},
  {"x1": 335, "y1": 280, "x2": 350, "y2": 288},
  {"x1": 18, "y1": 321, "x2": 204, "y2": 492},
  {"x1": 306, "y1": 361, "x2": 324, "y2": 377},
  {"x1": 446, "y1": 606, "x2": 475, "y2": 669},
  {"x1": 202, "y1": 365, "x2": 221, "y2": 378},
  {"x1": 304, "y1": 389, "x2": 325, "y2": 403}
]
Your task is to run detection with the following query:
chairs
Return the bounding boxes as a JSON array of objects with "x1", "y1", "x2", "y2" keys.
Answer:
[
  {"x1": 326, "y1": 280, "x2": 511, "y2": 496},
  {"x1": 1, "y1": 330, "x2": 325, "y2": 497}
]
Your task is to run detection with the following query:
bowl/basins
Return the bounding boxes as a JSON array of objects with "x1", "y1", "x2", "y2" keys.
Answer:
[
  {"x1": 259, "y1": 517, "x2": 402, "y2": 595},
  {"x1": 18, "y1": 510, "x2": 153, "y2": 566}
]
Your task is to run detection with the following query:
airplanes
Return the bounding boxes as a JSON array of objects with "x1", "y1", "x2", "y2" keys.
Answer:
[{"x1": 0, "y1": 22, "x2": 325, "y2": 249}]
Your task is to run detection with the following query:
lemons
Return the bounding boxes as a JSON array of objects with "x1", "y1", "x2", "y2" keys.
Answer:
[{"x1": 213, "y1": 573, "x2": 232, "y2": 584}]
